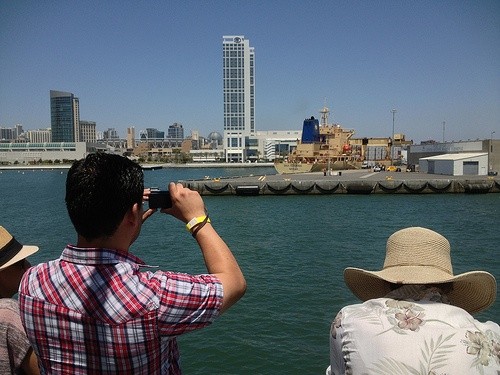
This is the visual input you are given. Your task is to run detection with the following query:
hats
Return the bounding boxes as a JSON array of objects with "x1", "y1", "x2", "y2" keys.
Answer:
[
  {"x1": 0, "y1": 224, "x2": 39, "y2": 271},
  {"x1": 343, "y1": 225, "x2": 498, "y2": 316}
]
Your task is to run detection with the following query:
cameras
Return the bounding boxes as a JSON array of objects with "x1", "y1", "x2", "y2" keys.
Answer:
[{"x1": 149, "y1": 190, "x2": 173, "y2": 209}]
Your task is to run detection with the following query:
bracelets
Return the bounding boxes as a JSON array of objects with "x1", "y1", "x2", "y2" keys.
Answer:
[{"x1": 186, "y1": 215, "x2": 211, "y2": 233}]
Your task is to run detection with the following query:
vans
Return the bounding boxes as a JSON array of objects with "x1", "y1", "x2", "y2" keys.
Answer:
[
  {"x1": 396, "y1": 163, "x2": 415, "y2": 172},
  {"x1": 387, "y1": 166, "x2": 396, "y2": 172}
]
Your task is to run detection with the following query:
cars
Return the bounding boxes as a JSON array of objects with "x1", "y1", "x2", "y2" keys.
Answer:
[{"x1": 373, "y1": 164, "x2": 385, "y2": 172}]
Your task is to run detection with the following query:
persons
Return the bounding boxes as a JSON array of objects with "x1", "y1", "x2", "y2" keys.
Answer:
[
  {"x1": 18, "y1": 150, "x2": 247, "y2": 375},
  {"x1": 0, "y1": 224, "x2": 42, "y2": 375},
  {"x1": 324, "y1": 226, "x2": 500, "y2": 375}
]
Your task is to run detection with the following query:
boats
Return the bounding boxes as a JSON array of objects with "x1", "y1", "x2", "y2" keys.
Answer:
[{"x1": 273, "y1": 105, "x2": 362, "y2": 175}]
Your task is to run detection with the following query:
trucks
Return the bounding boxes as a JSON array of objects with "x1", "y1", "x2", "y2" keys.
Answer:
[{"x1": 361, "y1": 161, "x2": 375, "y2": 169}]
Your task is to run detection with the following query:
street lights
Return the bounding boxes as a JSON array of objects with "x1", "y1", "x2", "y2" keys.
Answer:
[{"x1": 390, "y1": 108, "x2": 398, "y2": 165}]
